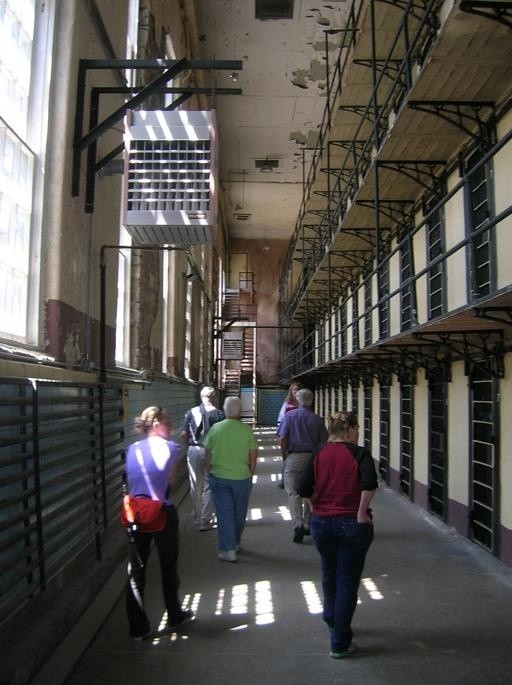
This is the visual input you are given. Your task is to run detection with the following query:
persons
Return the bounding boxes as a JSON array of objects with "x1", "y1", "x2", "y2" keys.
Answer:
[
  {"x1": 298, "y1": 411, "x2": 379, "y2": 658},
  {"x1": 276, "y1": 388, "x2": 329, "y2": 543},
  {"x1": 276, "y1": 382, "x2": 304, "y2": 427},
  {"x1": 121, "y1": 405, "x2": 194, "y2": 642},
  {"x1": 201, "y1": 396, "x2": 259, "y2": 562},
  {"x1": 180, "y1": 386, "x2": 226, "y2": 530}
]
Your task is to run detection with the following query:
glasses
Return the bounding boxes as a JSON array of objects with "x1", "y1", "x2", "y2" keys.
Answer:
[{"x1": 327, "y1": 411, "x2": 350, "y2": 422}]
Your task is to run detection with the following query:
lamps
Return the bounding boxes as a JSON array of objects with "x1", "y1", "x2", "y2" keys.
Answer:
[{"x1": 260, "y1": 164, "x2": 273, "y2": 174}]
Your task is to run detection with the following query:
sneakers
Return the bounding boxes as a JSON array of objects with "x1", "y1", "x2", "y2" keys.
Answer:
[
  {"x1": 129, "y1": 626, "x2": 154, "y2": 641},
  {"x1": 330, "y1": 641, "x2": 356, "y2": 658},
  {"x1": 198, "y1": 522, "x2": 212, "y2": 530},
  {"x1": 168, "y1": 610, "x2": 193, "y2": 629},
  {"x1": 293, "y1": 528, "x2": 304, "y2": 543},
  {"x1": 218, "y1": 550, "x2": 237, "y2": 562}
]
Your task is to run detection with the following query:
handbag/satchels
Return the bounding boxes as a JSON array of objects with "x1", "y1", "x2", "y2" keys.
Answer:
[{"x1": 120, "y1": 494, "x2": 166, "y2": 532}]
